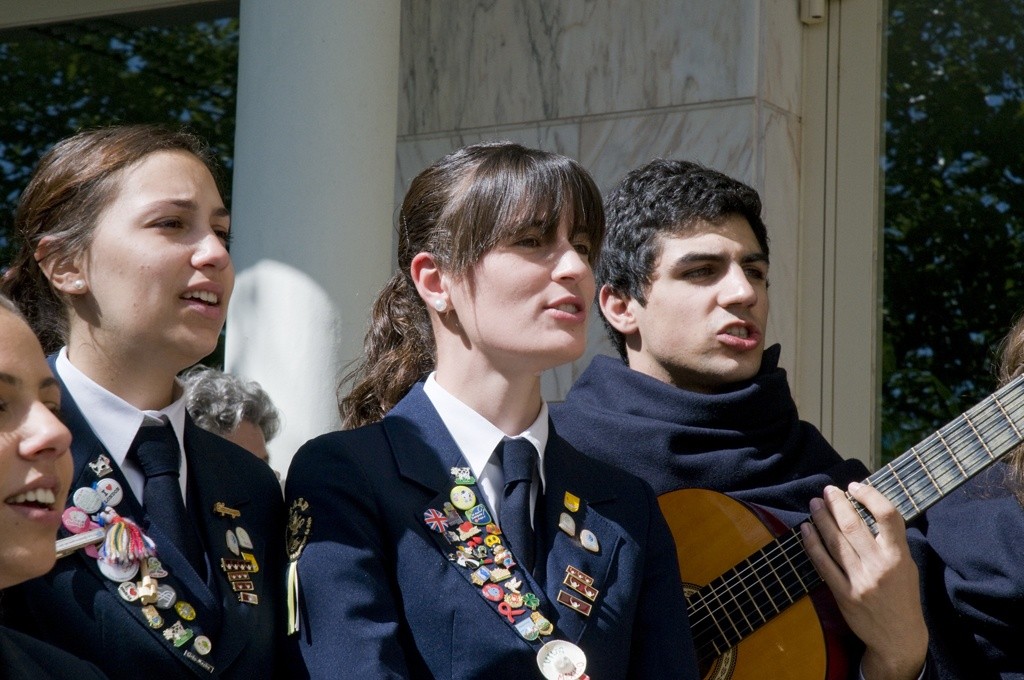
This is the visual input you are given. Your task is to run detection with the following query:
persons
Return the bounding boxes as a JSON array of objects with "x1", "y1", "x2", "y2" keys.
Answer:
[
  {"x1": 545, "y1": 156, "x2": 1004, "y2": 680},
  {"x1": 177, "y1": 363, "x2": 283, "y2": 489},
  {"x1": 923, "y1": 310, "x2": 1024, "y2": 680},
  {"x1": 0, "y1": 292, "x2": 110, "y2": 680},
  {"x1": 283, "y1": 136, "x2": 708, "y2": 680},
  {"x1": 0, "y1": 123, "x2": 286, "y2": 680}
]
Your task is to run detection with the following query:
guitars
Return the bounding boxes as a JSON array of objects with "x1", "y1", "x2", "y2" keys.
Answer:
[{"x1": 658, "y1": 376, "x2": 1023, "y2": 680}]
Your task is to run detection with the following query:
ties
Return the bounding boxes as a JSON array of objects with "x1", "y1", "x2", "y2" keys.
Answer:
[
  {"x1": 495, "y1": 439, "x2": 538, "y2": 575},
  {"x1": 126, "y1": 415, "x2": 209, "y2": 584}
]
